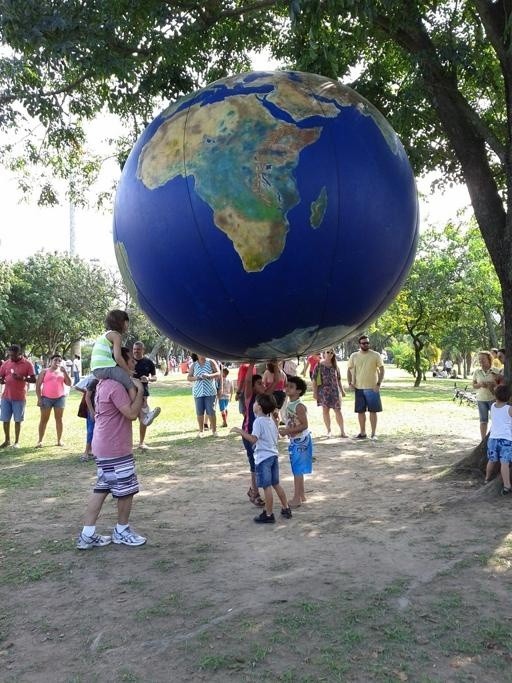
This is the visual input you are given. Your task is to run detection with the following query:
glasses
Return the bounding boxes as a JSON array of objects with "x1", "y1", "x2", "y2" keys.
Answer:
[{"x1": 360, "y1": 342, "x2": 370, "y2": 345}]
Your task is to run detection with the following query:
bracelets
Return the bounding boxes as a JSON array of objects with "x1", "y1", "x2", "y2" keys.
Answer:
[{"x1": 377, "y1": 383, "x2": 381, "y2": 386}]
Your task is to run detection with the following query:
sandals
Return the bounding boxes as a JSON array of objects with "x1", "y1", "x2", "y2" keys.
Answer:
[{"x1": 142, "y1": 407, "x2": 161, "y2": 426}]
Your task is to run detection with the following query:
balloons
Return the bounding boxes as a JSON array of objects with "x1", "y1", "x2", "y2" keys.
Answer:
[{"x1": 108, "y1": 65, "x2": 424, "y2": 370}]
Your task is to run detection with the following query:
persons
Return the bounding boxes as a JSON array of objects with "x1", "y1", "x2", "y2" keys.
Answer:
[
  {"x1": 75, "y1": 347, "x2": 148, "y2": 551},
  {"x1": 89, "y1": 306, "x2": 164, "y2": 428},
  {"x1": 232, "y1": 356, "x2": 279, "y2": 507},
  {"x1": 227, "y1": 391, "x2": 293, "y2": 524},
  {"x1": 347, "y1": 336, "x2": 385, "y2": 440},
  {"x1": 130, "y1": 341, "x2": 155, "y2": 448},
  {"x1": 281, "y1": 352, "x2": 323, "y2": 384},
  {"x1": 311, "y1": 347, "x2": 349, "y2": 437},
  {"x1": 157, "y1": 353, "x2": 193, "y2": 376},
  {"x1": 272, "y1": 372, "x2": 315, "y2": 510},
  {"x1": 480, "y1": 381, "x2": 510, "y2": 496},
  {"x1": 74, "y1": 362, "x2": 98, "y2": 462},
  {"x1": 2, "y1": 345, "x2": 82, "y2": 448},
  {"x1": 433, "y1": 358, "x2": 457, "y2": 379},
  {"x1": 188, "y1": 353, "x2": 232, "y2": 438},
  {"x1": 473, "y1": 348, "x2": 506, "y2": 440}
]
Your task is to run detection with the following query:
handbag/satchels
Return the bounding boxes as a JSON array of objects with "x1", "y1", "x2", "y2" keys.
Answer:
[{"x1": 316, "y1": 368, "x2": 322, "y2": 386}]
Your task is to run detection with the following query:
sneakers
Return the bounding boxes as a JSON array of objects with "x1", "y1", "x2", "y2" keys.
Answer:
[
  {"x1": 204, "y1": 424, "x2": 208, "y2": 431},
  {"x1": 247, "y1": 487, "x2": 292, "y2": 523},
  {"x1": 111, "y1": 526, "x2": 147, "y2": 546},
  {"x1": 76, "y1": 532, "x2": 112, "y2": 550}
]
